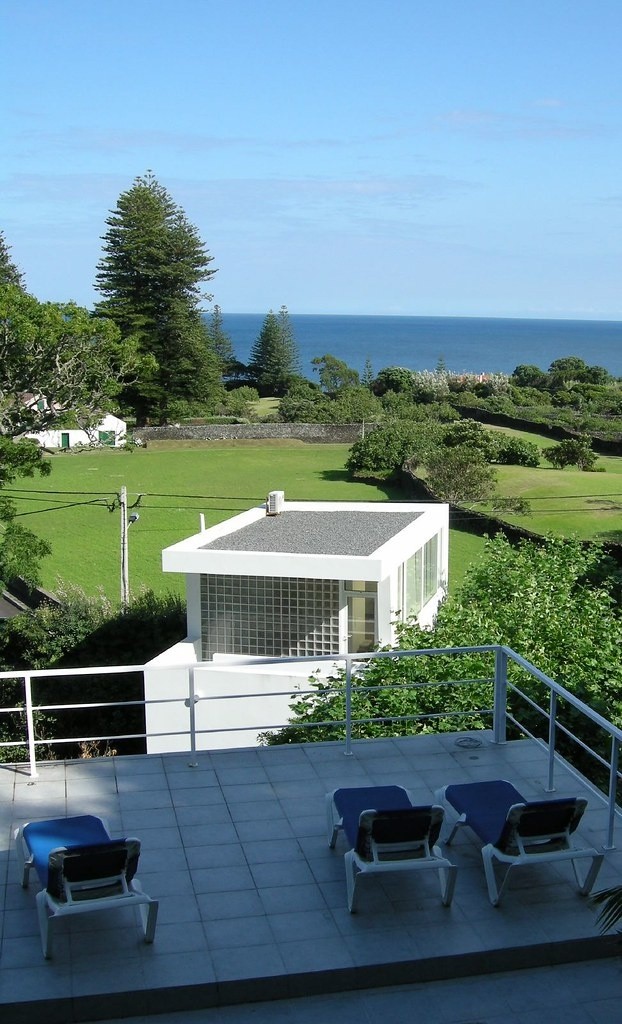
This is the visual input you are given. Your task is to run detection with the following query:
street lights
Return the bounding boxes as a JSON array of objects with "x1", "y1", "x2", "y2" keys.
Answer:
[{"x1": 121, "y1": 510, "x2": 139, "y2": 615}]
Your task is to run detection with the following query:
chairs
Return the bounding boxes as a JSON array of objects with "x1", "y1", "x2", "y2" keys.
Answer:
[
  {"x1": 433, "y1": 779, "x2": 605, "y2": 908},
  {"x1": 12, "y1": 813, "x2": 159, "y2": 961},
  {"x1": 323, "y1": 783, "x2": 459, "y2": 915}
]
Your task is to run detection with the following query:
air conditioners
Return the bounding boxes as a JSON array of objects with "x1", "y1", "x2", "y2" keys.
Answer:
[{"x1": 266, "y1": 490, "x2": 285, "y2": 516}]
